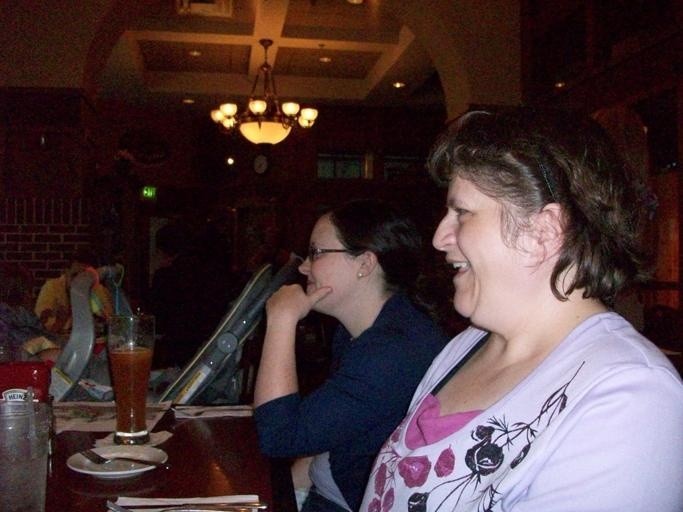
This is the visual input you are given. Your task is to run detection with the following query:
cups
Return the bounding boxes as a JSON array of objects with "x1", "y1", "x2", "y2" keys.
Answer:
[
  {"x1": 0, "y1": 400, "x2": 49, "y2": 512},
  {"x1": 107, "y1": 315, "x2": 155, "y2": 447}
]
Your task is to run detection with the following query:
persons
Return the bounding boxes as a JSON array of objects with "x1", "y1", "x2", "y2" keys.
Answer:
[
  {"x1": 252, "y1": 198, "x2": 445, "y2": 512},
  {"x1": 35, "y1": 242, "x2": 111, "y2": 333},
  {"x1": 355, "y1": 103, "x2": 683, "y2": 512}
]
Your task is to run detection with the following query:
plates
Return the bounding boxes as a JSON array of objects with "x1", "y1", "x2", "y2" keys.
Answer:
[{"x1": 66, "y1": 445, "x2": 168, "y2": 479}]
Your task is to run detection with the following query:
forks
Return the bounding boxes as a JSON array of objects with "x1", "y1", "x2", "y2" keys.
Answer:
[
  {"x1": 76, "y1": 446, "x2": 173, "y2": 470},
  {"x1": 106, "y1": 501, "x2": 253, "y2": 512}
]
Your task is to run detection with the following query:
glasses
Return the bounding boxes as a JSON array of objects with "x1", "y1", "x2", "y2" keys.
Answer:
[{"x1": 308, "y1": 246, "x2": 352, "y2": 261}]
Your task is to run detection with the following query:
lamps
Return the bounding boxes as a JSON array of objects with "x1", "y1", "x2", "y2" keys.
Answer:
[{"x1": 209, "y1": 38, "x2": 319, "y2": 153}]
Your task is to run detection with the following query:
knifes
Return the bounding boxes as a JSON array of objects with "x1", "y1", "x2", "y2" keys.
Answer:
[{"x1": 104, "y1": 501, "x2": 269, "y2": 509}]
